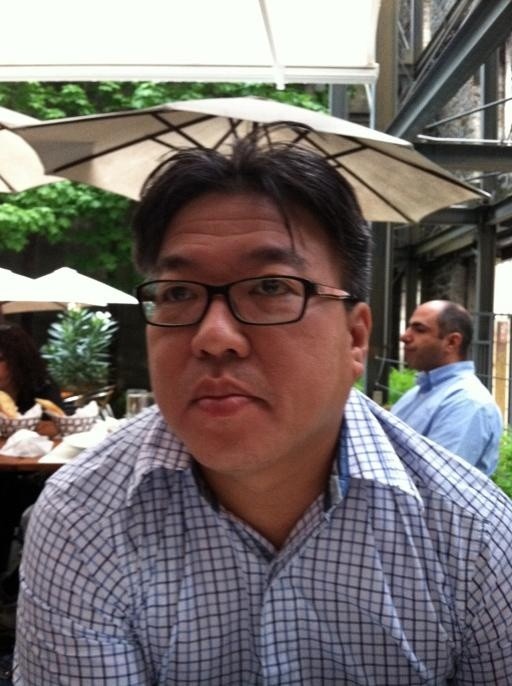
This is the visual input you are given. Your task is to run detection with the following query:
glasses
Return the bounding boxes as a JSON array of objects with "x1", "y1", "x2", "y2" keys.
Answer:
[{"x1": 136, "y1": 276, "x2": 357, "y2": 328}]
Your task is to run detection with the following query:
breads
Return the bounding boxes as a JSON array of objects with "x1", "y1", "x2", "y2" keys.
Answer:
[
  {"x1": 0, "y1": 390, "x2": 18, "y2": 419},
  {"x1": 35, "y1": 398, "x2": 65, "y2": 415}
]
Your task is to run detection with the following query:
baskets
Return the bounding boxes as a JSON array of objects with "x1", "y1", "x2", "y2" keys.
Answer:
[
  {"x1": 53, "y1": 416, "x2": 94, "y2": 437},
  {"x1": 0, "y1": 415, "x2": 43, "y2": 439}
]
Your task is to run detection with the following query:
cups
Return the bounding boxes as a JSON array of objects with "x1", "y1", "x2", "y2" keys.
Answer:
[{"x1": 124, "y1": 387, "x2": 148, "y2": 415}]
